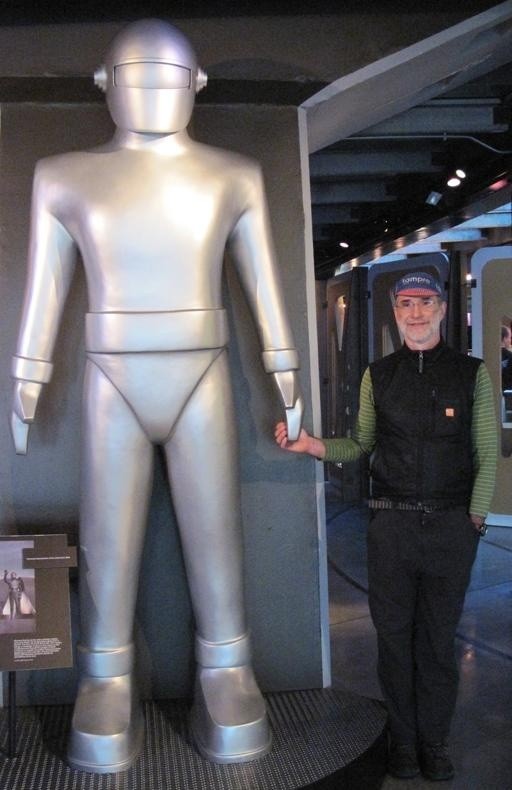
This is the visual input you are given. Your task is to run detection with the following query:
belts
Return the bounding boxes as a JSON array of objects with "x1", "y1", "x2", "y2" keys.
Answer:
[{"x1": 363, "y1": 496, "x2": 448, "y2": 513}]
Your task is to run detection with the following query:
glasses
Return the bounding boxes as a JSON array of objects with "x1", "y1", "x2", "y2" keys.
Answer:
[{"x1": 394, "y1": 298, "x2": 441, "y2": 309}]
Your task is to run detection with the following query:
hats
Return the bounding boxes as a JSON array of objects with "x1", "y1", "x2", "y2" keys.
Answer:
[{"x1": 388, "y1": 271, "x2": 445, "y2": 299}]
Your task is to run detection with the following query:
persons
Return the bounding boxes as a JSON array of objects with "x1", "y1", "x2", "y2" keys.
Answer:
[
  {"x1": 499, "y1": 323, "x2": 512, "y2": 391},
  {"x1": 11, "y1": 17, "x2": 306, "y2": 773},
  {"x1": 270, "y1": 271, "x2": 501, "y2": 782},
  {"x1": 3, "y1": 569, "x2": 28, "y2": 620}
]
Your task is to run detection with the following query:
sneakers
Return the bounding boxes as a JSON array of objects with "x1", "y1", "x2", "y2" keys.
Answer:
[
  {"x1": 386, "y1": 742, "x2": 420, "y2": 780},
  {"x1": 415, "y1": 740, "x2": 456, "y2": 782}
]
